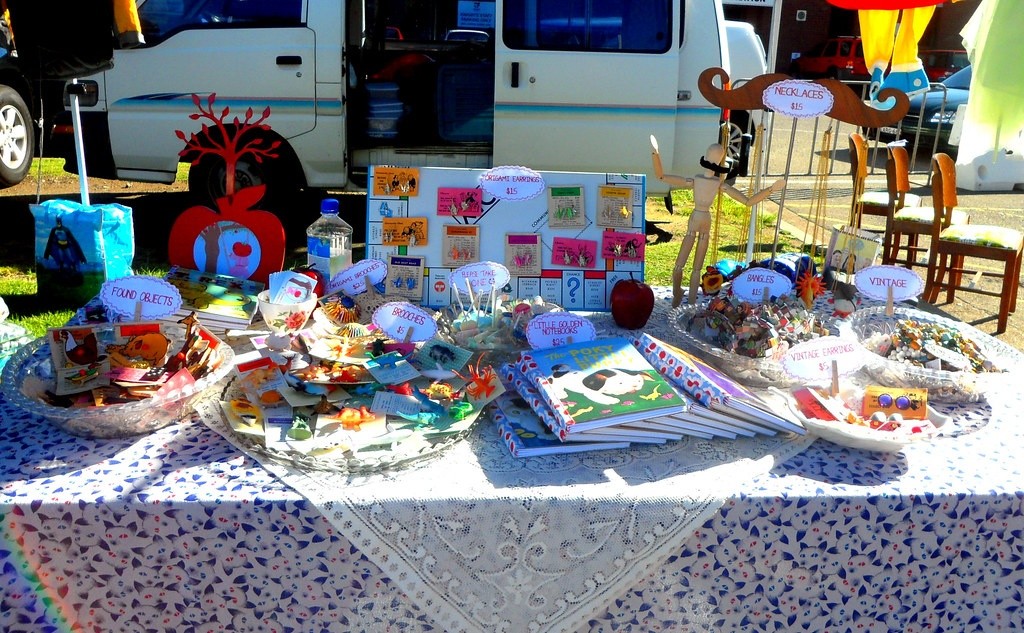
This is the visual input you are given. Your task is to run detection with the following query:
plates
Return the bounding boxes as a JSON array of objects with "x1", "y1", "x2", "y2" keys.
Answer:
[
  {"x1": 439, "y1": 295, "x2": 571, "y2": 362},
  {"x1": 667, "y1": 299, "x2": 1023, "y2": 452},
  {"x1": 220, "y1": 373, "x2": 488, "y2": 474},
  {"x1": 0, "y1": 320, "x2": 236, "y2": 435}
]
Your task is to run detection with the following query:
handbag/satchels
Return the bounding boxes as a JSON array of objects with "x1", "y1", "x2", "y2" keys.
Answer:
[{"x1": 28, "y1": 199, "x2": 136, "y2": 282}]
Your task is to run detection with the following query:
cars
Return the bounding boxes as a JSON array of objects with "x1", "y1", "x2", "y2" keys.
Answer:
[
  {"x1": 861, "y1": 65, "x2": 971, "y2": 156},
  {"x1": 0, "y1": 0, "x2": 114, "y2": 185}
]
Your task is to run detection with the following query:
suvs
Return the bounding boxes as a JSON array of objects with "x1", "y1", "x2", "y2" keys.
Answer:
[
  {"x1": 793, "y1": 35, "x2": 970, "y2": 83},
  {"x1": 719, "y1": 20, "x2": 768, "y2": 182}
]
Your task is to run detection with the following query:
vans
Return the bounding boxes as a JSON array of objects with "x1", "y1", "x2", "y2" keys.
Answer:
[{"x1": 53, "y1": 0, "x2": 730, "y2": 196}]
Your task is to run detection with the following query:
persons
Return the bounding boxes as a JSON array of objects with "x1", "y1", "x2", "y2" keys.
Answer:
[{"x1": 649, "y1": 134, "x2": 785, "y2": 308}]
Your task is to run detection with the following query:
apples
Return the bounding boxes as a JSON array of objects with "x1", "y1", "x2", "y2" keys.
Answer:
[{"x1": 610, "y1": 271, "x2": 654, "y2": 329}]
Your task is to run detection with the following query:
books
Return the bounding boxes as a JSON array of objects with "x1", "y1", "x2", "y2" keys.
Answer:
[
  {"x1": 160, "y1": 267, "x2": 266, "y2": 341},
  {"x1": 488, "y1": 332, "x2": 807, "y2": 458}
]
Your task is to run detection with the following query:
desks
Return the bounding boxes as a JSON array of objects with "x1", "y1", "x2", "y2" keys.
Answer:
[{"x1": 0, "y1": 312, "x2": 1024, "y2": 633}]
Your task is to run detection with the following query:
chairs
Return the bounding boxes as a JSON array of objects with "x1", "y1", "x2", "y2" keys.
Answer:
[
  {"x1": 882, "y1": 145, "x2": 970, "y2": 305},
  {"x1": 922, "y1": 152, "x2": 1024, "y2": 333},
  {"x1": 848, "y1": 133, "x2": 921, "y2": 270}
]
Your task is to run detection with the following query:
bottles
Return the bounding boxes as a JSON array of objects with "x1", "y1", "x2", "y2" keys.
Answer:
[{"x1": 308, "y1": 198, "x2": 353, "y2": 300}]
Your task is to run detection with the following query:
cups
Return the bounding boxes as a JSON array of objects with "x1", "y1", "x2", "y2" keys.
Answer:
[{"x1": 258, "y1": 287, "x2": 318, "y2": 336}]
